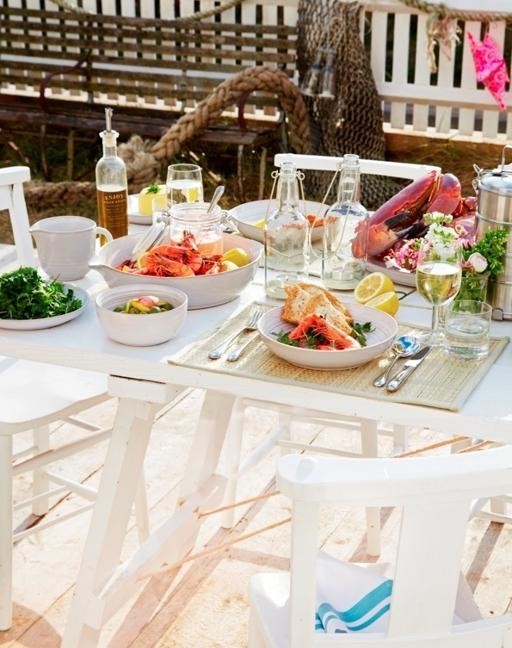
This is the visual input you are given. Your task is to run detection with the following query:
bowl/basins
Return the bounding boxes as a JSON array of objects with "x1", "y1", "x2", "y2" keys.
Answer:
[
  {"x1": 88, "y1": 231, "x2": 264, "y2": 309},
  {"x1": 96, "y1": 283, "x2": 188, "y2": 346}
]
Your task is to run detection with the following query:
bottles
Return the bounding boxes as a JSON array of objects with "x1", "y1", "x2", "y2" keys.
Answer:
[
  {"x1": 322, "y1": 154, "x2": 369, "y2": 290},
  {"x1": 298, "y1": 49, "x2": 324, "y2": 98},
  {"x1": 319, "y1": 48, "x2": 337, "y2": 98},
  {"x1": 264, "y1": 161, "x2": 309, "y2": 299},
  {"x1": 94, "y1": 132, "x2": 128, "y2": 247}
]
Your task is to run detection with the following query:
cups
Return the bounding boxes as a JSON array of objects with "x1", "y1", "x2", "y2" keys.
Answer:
[
  {"x1": 29, "y1": 216, "x2": 114, "y2": 280},
  {"x1": 441, "y1": 299, "x2": 491, "y2": 360},
  {"x1": 167, "y1": 202, "x2": 224, "y2": 260},
  {"x1": 166, "y1": 164, "x2": 203, "y2": 209}
]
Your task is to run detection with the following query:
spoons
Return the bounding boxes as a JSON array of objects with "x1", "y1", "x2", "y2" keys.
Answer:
[{"x1": 373, "y1": 336, "x2": 420, "y2": 387}]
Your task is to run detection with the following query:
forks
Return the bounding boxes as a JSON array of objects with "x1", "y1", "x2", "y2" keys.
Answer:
[{"x1": 210, "y1": 311, "x2": 264, "y2": 361}]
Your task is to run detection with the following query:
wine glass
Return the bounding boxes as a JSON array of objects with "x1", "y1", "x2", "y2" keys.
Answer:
[{"x1": 415, "y1": 238, "x2": 464, "y2": 346}]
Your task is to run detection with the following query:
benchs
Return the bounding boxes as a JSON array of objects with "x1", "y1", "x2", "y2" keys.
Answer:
[{"x1": 1, "y1": 6, "x2": 300, "y2": 204}]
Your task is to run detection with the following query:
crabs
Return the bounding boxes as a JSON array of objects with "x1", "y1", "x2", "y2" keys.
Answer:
[{"x1": 351, "y1": 170, "x2": 478, "y2": 263}]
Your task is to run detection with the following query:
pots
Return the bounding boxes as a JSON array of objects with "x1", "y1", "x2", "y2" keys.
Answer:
[{"x1": 471, "y1": 145, "x2": 512, "y2": 324}]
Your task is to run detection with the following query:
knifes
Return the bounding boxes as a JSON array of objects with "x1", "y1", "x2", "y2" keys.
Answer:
[{"x1": 386, "y1": 343, "x2": 434, "y2": 396}]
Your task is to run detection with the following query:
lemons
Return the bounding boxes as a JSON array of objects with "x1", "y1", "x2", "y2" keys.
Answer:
[
  {"x1": 354, "y1": 271, "x2": 395, "y2": 301},
  {"x1": 364, "y1": 290, "x2": 398, "y2": 315}
]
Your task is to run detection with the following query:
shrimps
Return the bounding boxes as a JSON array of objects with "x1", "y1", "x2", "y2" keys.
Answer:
[
  {"x1": 146, "y1": 245, "x2": 200, "y2": 263},
  {"x1": 178, "y1": 229, "x2": 202, "y2": 271},
  {"x1": 147, "y1": 251, "x2": 194, "y2": 277},
  {"x1": 309, "y1": 314, "x2": 361, "y2": 351}
]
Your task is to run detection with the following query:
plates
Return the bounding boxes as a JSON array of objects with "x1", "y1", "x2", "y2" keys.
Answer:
[
  {"x1": 229, "y1": 199, "x2": 342, "y2": 244},
  {"x1": 257, "y1": 303, "x2": 398, "y2": 370},
  {"x1": 0, "y1": 282, "x2": 90, "y2": 329},
  {"x1": 339, "y1": 243, "x2": 420, "y2": 287}
]
